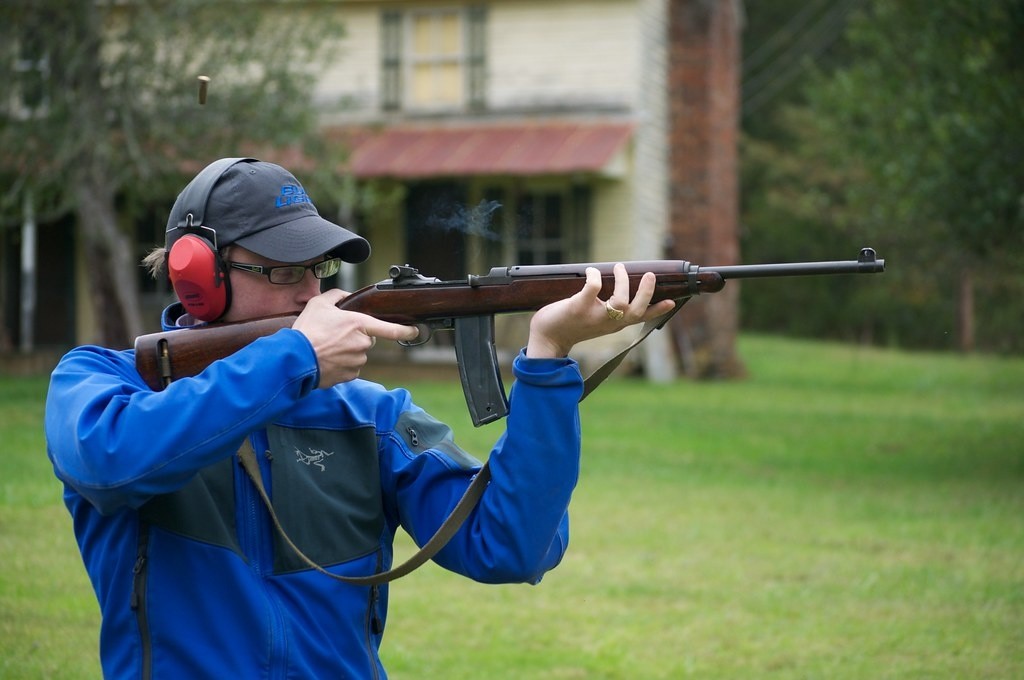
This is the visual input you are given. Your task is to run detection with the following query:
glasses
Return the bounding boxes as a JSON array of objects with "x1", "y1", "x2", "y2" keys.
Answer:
[{"x1": 225, "y1": 258, "x2": 342, "y2": 284}]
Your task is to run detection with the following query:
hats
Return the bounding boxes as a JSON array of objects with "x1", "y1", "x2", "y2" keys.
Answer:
[{"x1": 165, "y1": 157, "x2": 370, "y2": 264}]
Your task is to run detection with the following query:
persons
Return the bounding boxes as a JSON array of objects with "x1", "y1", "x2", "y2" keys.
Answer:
[{"x1": 45, "y1": 155, "x2": 675, "y2": 680}]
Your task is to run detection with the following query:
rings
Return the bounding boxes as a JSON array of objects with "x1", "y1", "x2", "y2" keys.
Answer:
[{"x1": 605, "y1": 299, "x2": 624, "y2": 322}]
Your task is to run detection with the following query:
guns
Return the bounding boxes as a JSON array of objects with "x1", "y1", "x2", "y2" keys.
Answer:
[{"x1": 133, "y1": 246, "x2": 889, "y2": 429}]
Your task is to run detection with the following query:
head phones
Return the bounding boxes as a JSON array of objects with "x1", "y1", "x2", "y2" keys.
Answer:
[{"x1": 165, "y1": 158, "x2": 265, "y2": 323}]
